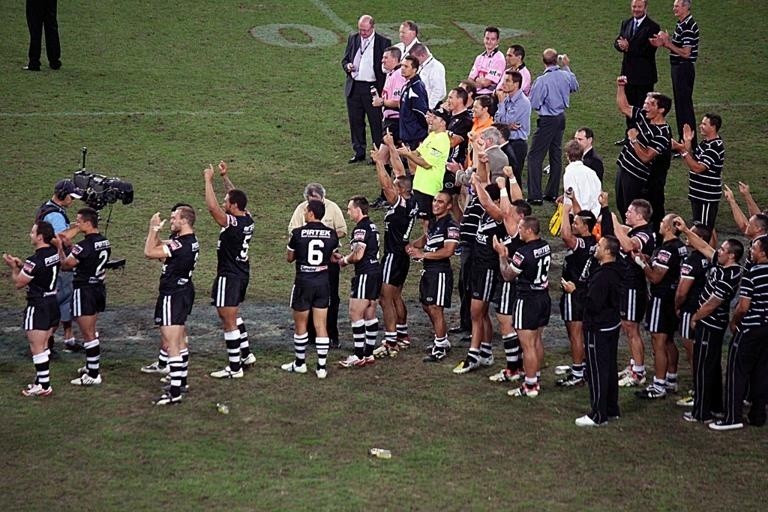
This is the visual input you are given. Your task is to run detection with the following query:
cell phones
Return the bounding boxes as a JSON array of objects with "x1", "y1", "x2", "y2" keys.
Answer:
[{"x1": 350, "y1": 65, "x2": 356, "y2": 72}]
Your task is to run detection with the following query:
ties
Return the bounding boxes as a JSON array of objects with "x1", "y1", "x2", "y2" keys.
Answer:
[
  {"x1": 633, "y1": 21, "x2": 638, "y2": 35},
  {"x1": 351, "y1": 39, "x2": 368, "y2": 78}
]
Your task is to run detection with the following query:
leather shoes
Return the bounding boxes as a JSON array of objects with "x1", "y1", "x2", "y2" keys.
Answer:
[
  {"x1": 525, "y1": 198, "x2": 543, "y2": 206},
  {"x1": 542, "y1": 194, "x2": 556, "y2": 203},
  {"x1": 348, "y1": 155, "x2": 365, "y2": 163},
  {"x1": 614, "y1": 138, "x2": 629, "y2": 145}
]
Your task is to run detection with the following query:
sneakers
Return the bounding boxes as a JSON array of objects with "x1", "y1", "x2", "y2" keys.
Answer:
[
  {"x1": 210, "y1": 368, "x2": 244, "y2": 379},
  {"x1": 369, "y1": 195, "x2": 391, "y2": 209},
  {"x1": 575, "y1": 415, "x2": 608, "y2": 427},
  {"x1": 62, "y1": 340, "x2": 102, "y2": 385},
  {"x1": 617, "y1": 365, "x2": 744, "y2": 430},
  {"x1": 280, "y1": 360, "x2": 308, "y2": 373},
  {"x1": 141, "y1": 361, "x2": 190, "y2": 405},
  {"x1": 225, "y1": 353, "x2": 256, "y2": 371},
  {"x1": 422, "y1": 326, "x2": 588, "y2": 398},
  {"x1": 21, "y1": 384, "x2": 53, "y2": 397},
  {"x1": 315, "y1": 368, "x2": 328, "y2": 378},
  {"x1": 339, "y1": 336, "x2": 411, "y2": 369}
]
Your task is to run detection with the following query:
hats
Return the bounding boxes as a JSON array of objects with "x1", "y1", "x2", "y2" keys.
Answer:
[
  {"x1": 432, "y1": 107, "x2": 451, "y2": 123},
  {"x1": 54, "y1": 180, "x2": 84, "y2": 199}
]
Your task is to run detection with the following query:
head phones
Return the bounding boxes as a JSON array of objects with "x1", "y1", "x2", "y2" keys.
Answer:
[{"x1": 58, "y1": 179, "x2": 67, "y2": 201}]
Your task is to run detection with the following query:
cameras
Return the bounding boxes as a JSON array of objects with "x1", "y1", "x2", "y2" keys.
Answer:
[{"x1": 558, "y1": 54, "x2": 566, "y2": 63}]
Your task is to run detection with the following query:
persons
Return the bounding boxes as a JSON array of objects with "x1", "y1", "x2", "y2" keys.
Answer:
[
  {"x1": 203, "y1": 161, "x2": 258, "y2": 379},
  {"x1": 2, "y1": 221, "x2": 61, "y2": 398},
  {"x1": 22, "y1": 2, "x2": 62, "y2": 72},
  {"x1": 35, "y1": 179, "x2": 83, "y2": 352},
  {"x1": 282, "y1": 14, "x2": 768, "y2": 430},
  {"x1": 651, "y1": 2, "x2": 700, "y2": 155},
  {"x1": 613, "y1": 2, "x2": 660, "y2": 145},
  {"x1": 57, "y1": 208, "x2": 112, "y2": 386},
  {"x1": 140, "y1": 202, "x2": 199, "y2": 406}
]
